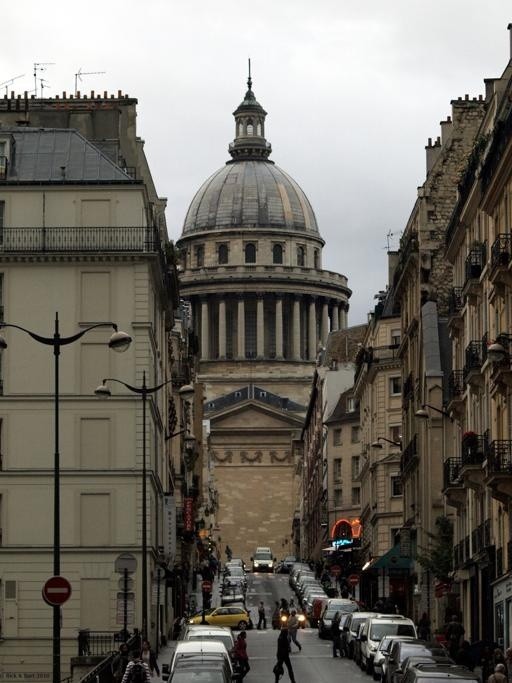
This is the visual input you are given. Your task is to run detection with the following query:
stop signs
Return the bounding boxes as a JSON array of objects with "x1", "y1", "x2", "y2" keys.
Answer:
[{"x1": 42, "y1": 576, "x2": 72, "y2": 605}]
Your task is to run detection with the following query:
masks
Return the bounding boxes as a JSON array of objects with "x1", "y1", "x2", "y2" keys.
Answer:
[{"x1": 133, "y1": 658, "x2": 140, "y2": 662}]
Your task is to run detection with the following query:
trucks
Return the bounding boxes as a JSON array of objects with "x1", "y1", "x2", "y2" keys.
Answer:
[{"x1": 251, "y1": 546, "x2": 277, "y2": 572}]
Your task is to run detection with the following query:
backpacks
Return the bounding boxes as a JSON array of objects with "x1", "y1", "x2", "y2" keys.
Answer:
[{"x1": 131, "y1": 661, "x2": 146, "y2": 683}]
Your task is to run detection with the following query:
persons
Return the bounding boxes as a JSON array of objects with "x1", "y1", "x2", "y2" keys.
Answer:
[
  {"x1": 257, "y1": 601, "x2": 266, "y2": 630},
  {"x1": 271, "y1": 598, "x2": 306, "y2": 631},
  {"x1": 305, "y1": 555, "x2": 351, "y2": 600},
  {"x1": 275, "y1": 628, "x2": 296, "y2": 683},
  {"x1": 287, "y1": 610, "x2": 301, "y2": 652},
  {"x1": 331, "y1": 612, "x2": 346, "y2": 658},
  {"x1": 445, "y1": 614, "x2": 512, "y2": 683},
  {"x1": 235, "y1": 632, "x2": 250, "y2": 683},
  {"x1": 111, "y1": 628, "x2": 160, "y2": 683},
  {"x1": 273, "y1": 557, "x2": 281, "y2": 574},
  {"x1": 225, "y1": 545, "x2": 232, "y2": 560},
  {"x1": 172, "y1": 613, "x2": 187, "y2": 641},
  {"x1": 371, "y1": 597, "x2": 397, "y2": 614},
  {"x1": 417, "y1": 613, "x2": 431, "y2": 640},
  {"x1": 197, "y1": 549, "x2": 222, "y2": 585}
]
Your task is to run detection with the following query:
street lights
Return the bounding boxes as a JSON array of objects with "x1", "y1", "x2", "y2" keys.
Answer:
[
  {"x1": 93, "y1": 371, "x2": 198, "y2": 649},
  {"x1": 1, "y1": 313, "x2": 137, "y2": 682}
]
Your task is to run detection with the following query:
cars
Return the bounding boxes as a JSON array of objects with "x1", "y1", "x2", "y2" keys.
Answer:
[
  {"x1": 216, "y1": 555, "x2": 247, "y2": 602},
  {"x1": 162, "y1": 601, "x2": 253, "y2": 683},
  {"x1": 273, "y1": 606, "x2": 307, "y2": 628},
  {"x1": 282, "y1": 556, "x2": 480, "y2": 681}
]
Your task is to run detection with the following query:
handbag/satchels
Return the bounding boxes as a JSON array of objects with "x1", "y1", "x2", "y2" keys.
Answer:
[{"x1": 273, "y1": 665, "x2": 284, "y2": 675}]
